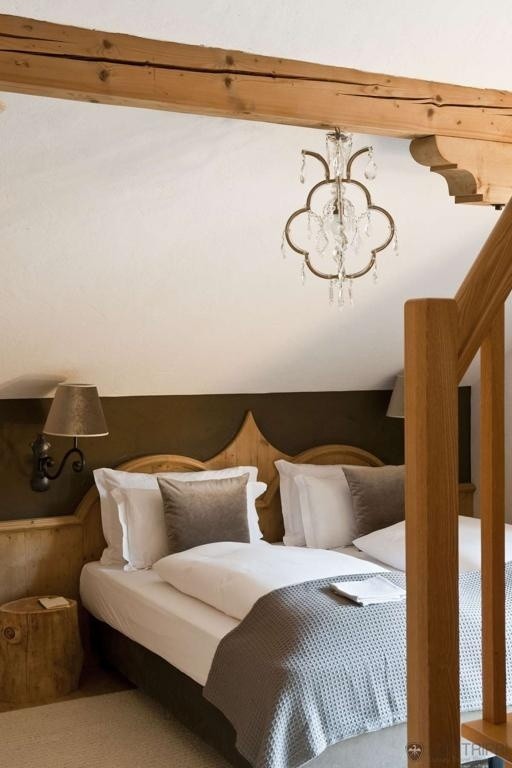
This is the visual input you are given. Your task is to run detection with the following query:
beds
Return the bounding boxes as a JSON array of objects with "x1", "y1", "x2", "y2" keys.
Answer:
[{"x1": 79, "y1": 410, "x2": 511, "y2": 768}]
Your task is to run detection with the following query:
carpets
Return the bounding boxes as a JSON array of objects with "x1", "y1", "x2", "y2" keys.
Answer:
[{"x1": 0, "y1": 690, "x2": 233, "y2": 768}]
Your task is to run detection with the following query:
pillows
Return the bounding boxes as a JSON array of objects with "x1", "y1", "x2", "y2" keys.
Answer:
[
  {"x1": 156, "y1": 473, "x2": 250, "y2": 552},
  {"x1": 342, "y1": 466, "x2": 405, "y2": 551},
  {"x1": 92, "y1": 466, "x2": 258, "y2": 568},
  {"x1": 293, "y1": 475, "x2": 359, "y2": 551},
  {"x1": 109, "y1": 482, "x2": 268, "y2": 573},
  {"x1": 273, "y1": 459, "x2": 351, "y2": 547}
]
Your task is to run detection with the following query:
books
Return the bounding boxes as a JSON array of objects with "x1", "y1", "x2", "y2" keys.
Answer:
[{"x1": 37, "y1": 594, "x2": 70, "y2": 609}]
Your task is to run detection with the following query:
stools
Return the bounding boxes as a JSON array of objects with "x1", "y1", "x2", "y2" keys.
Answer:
[{"x1": 0, "y1": 596, "x2": 84, "y2": 703}]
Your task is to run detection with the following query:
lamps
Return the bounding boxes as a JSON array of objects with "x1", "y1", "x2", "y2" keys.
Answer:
[
  {"x1": 30, "y1": 384, "x2": 110, "y2": 492},
  {"x1": 279, "y1": 126, "x2": 400, "y2": 306},
  {"x1": 386, "y1": 372, "x2": 406, "y2": 418}
]
